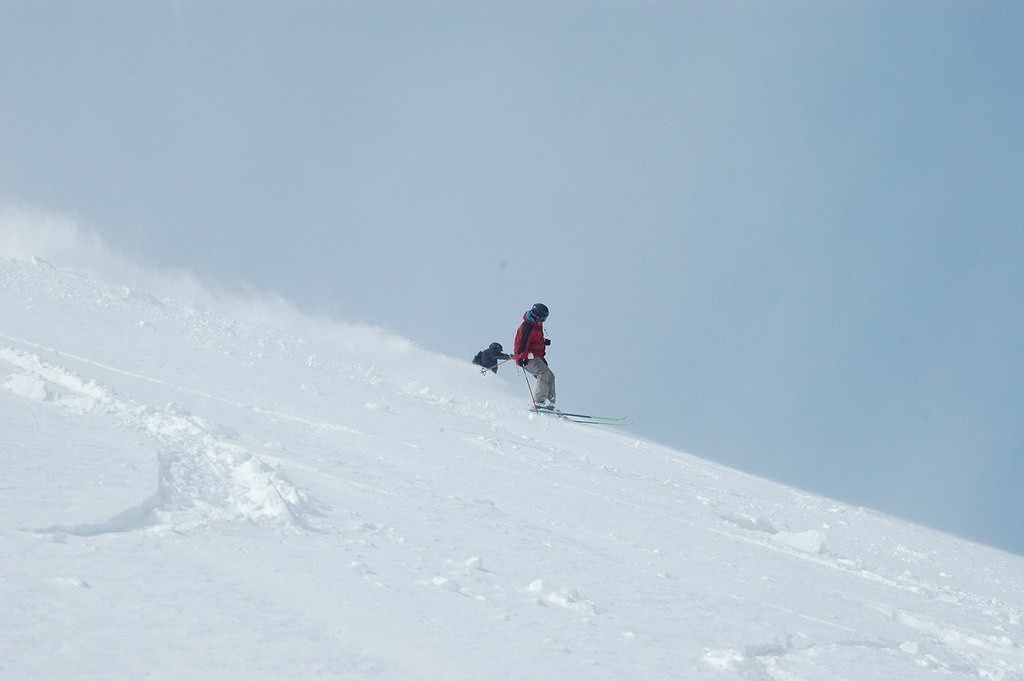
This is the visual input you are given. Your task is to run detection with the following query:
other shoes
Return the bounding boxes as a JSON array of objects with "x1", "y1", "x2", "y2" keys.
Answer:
[{"x1": 532, "y1": 402, "x2": 554, "y2": 410}]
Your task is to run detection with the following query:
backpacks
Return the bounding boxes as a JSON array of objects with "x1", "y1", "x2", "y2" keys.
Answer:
[{"x1": 472, "y1": 351, "x2": 483, "y2": 365}]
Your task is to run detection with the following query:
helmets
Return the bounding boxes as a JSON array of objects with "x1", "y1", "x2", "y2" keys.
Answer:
[
  {"x1": 489, "y1": 342, "x2": 502, "y2": 350},
  {"x1": 531, "y1": 303, "x2": 549, "y2": 322}
]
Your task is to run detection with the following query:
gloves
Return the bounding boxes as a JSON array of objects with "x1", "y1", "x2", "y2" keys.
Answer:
[
  {"x1": 518, "y1": 358, "x2": 528, "y2": 367},
  {"x1": 544, "y1": 339, "x2": 551, "y2": 346}
]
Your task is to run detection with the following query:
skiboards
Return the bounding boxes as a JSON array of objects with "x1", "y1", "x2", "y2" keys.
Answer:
[{"x1": 529, "y1": 407, "x2": 634, "y2": 428}]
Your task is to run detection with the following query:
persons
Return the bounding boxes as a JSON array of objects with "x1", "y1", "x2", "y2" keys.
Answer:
[
  {"x1": 513, "y1": 303, "x2": 561, "y2": 411},
  {"x1": 472, "y1": 342, "x2": 513, "y2": 373}
]
